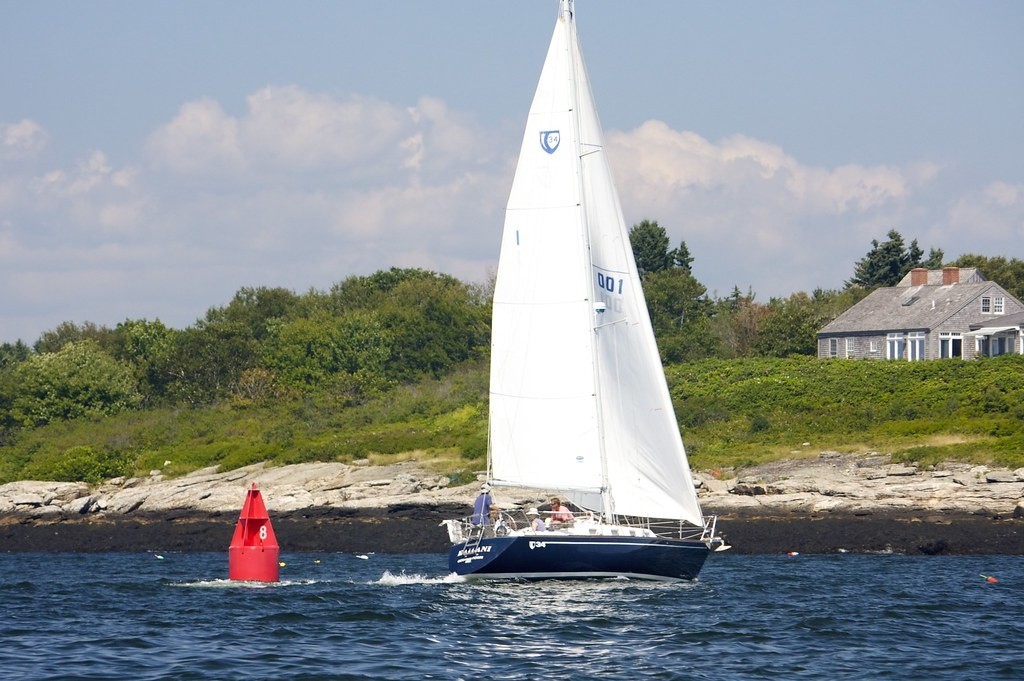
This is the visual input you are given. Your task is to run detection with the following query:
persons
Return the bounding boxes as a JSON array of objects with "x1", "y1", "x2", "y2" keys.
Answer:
[
  {"x1": 549, "y1": 498, "x2": 574, "y2": 523},
  {"x1": 525, "y1": 509, "x2": 545, "y2": 532},
  {"x1": 471, "y1": 483, "x2": 499, "y2": 537}
]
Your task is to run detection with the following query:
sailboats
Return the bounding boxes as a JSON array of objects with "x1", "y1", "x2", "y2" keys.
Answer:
[{"x1": 438, "y1": 0, "x2": 731, "y2": 580}]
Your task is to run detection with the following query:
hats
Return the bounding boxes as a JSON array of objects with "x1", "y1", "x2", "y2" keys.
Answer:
[
  {"x1": 526, "y1": 508, "x2": 541, "y2": 515},
  {"x1": 481, "y1": 484, "x2": 491, "y2": 493}
]
